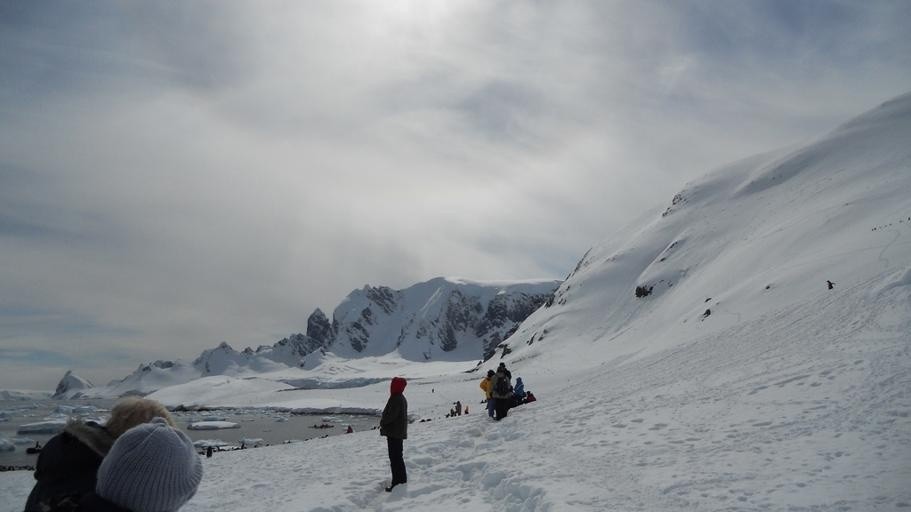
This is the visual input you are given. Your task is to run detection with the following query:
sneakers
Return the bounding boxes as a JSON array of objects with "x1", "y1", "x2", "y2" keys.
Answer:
[{"x1": 385, "y1": 484, "x2": 394, "y2": 492}]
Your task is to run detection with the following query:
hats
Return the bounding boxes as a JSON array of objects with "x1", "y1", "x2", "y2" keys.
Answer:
[{"x1": 95, "y1": 396, "x2": 203, "y2": 512}]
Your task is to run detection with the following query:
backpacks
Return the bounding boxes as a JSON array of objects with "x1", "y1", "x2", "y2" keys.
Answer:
[{"x1": 493, "y1": 373, "x2": 509, "y2": 396}]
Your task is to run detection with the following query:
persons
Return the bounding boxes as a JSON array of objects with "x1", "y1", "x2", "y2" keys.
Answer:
[
  {"x1": 446, "y1": 401, "x2": 469, "y2": 417},
  {"x1": 23, "y1": 397, "x2": 203, "y2": 512},
  {"x1": 480, "y1": 362, "x2": 536, "y2": 420},
  {"x1": 379, "y1": 377, "x2": 407, "y2": 492}
]
